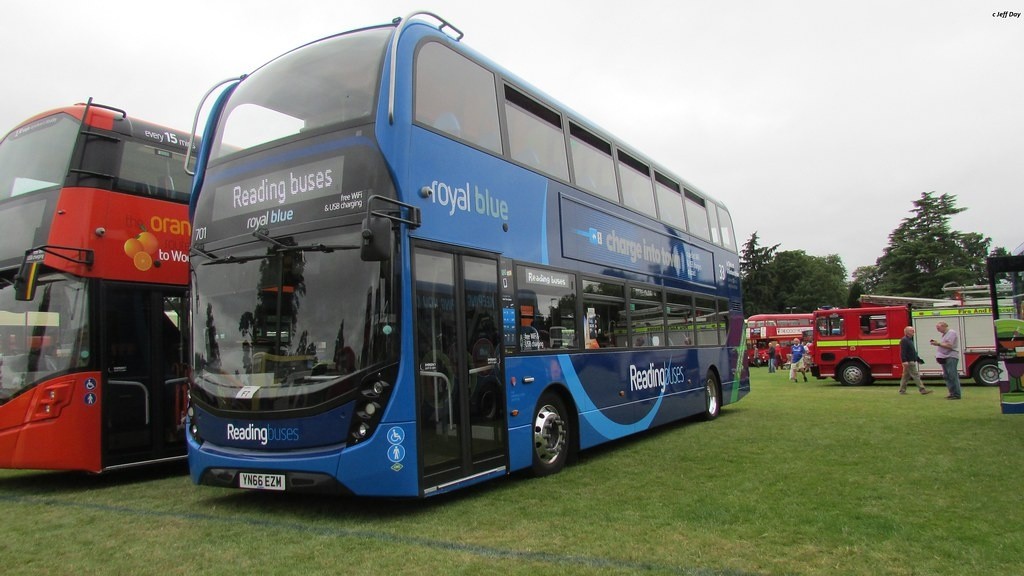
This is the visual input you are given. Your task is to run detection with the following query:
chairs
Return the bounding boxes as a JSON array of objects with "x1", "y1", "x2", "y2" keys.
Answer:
[
  {"x1": 156, "y1": 173, "x2": 174, "y2": 190},
  {"x1": 580, "y1": 174, "x2": 599, "y2": 193},
  {"x1": 434, "y1": 111, "x2": 461, "y2": 138},
  {"x1": 518, "y1": 147, "x2": 541, "y2": 169},
  {"x1": 661, "y1": 208, "x2": 672, "y2": 223}
]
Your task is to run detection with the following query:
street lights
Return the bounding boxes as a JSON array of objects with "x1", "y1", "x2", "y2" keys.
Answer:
[{"x1": 786, "y1": 307, "x2": 797, "y2": 314}]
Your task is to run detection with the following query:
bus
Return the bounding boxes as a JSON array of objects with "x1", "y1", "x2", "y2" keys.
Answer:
[
  {"x1": 183, "y1": 8, "x2": 751, "y2": 497},
  {"x1": 0, "y1": 96, "x2": 241, "y2": 474},
  {"x1": 745, "y1": 312, "x2": 813, "y2": 366}
]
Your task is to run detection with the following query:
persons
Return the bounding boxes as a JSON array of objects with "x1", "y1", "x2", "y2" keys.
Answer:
[
  {"x1": 931, "y1": 321, "x2": 961, "y2": 400},
  {"x1": 775, "y1": 344, "x2": 784, "y2": 371},
  {"x1": 339, "y1": 347, "x2": 357, "y2": 374},
  {"x1": 899, "y1": 326, "x2": 930, "y2": 394},
  {"x1": 791, "y1": 338, "x2": 807, "y2": 382},
  {"x1": 753, "y1": 345, "x2": 759, "y2": 368},
  {"x1": 802, "y1": 340, "x2": 814, "y2": 372},
  {"x1": 531, "y1": 320, "x2": 550, "y2": 350},
  {"x1": 764, "y1": 343, "x2": 776, "y2": 373},
  {"x1": 685, "y1": 337, "x2": 691, "y2": 345},
  {"x1": 634, "y1": 337, "x2": 645, "y2": 347}
]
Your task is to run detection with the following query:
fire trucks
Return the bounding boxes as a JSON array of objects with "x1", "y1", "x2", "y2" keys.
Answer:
[{"x1": 810, "y1": 281, "x2": 1018, "y2": 387}]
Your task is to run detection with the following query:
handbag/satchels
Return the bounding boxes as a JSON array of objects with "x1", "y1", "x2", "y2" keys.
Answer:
[
  {"x1": 936, "y1": 358, "x2": 947, "y2": 364},
  {"x1": 790, "y1": 369, "x2": 794, "y2": 379}
]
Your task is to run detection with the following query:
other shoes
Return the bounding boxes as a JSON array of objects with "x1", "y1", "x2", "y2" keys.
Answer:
[
  {"x1": 804, "y1": 377, "x2": 807, "y2": 382},
  {"x1": 944, "y1": 395, "x2": 961, "y2": 400},
  {"x1": 900, "y1": 390, "x2": 911, "y2": 395},
  {"x1": 795, "y1": 379, "x2": 798, "y2": 382},
  {"x1": 921, "y1": 389, "x2": 933, "y2": 395}
]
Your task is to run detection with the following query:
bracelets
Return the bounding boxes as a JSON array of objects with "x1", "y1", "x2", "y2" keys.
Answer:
[{"x1": 938, "y1": 342, "x2": 940, "y2": 346}]
